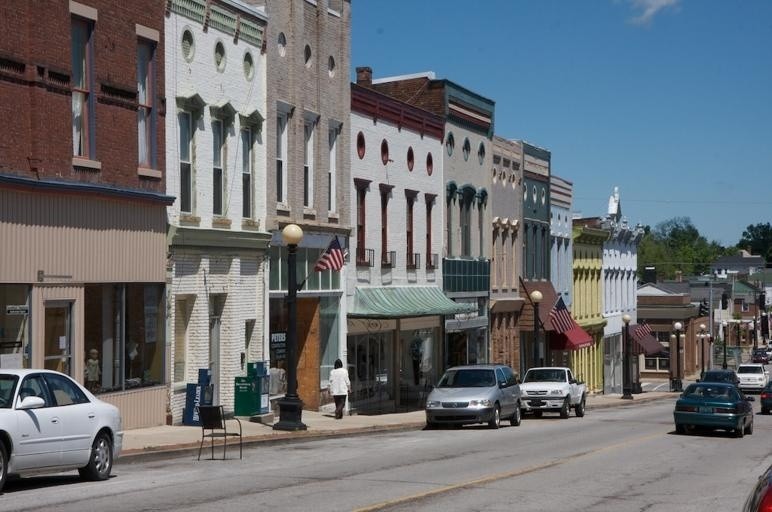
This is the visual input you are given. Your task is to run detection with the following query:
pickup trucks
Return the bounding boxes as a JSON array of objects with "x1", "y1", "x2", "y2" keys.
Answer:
[{"x1": 519, "y1": 367, "x2": 588, "y2": 418}]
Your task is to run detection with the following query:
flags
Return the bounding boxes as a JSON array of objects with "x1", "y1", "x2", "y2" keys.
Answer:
[
  {"x1": 313, "y1": 235, "x2": 345, "y2": 274},
  {"x1": 548, "y1": 295, "x2": 575, "y2": 336},
  {"x1": 631, "y1": 320, "x2": 652, "y2": 341}
]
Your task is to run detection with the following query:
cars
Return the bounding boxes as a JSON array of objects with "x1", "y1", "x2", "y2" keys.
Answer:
[
  {"x1": 674, "y1": 363, "x2": 772, "y2": 437},
  {"x1": 425, "y1": 365, "x2": 521, "y2": 429},
  {"x1": 0, "y1": 368, "x2": 123, "y2": 491},
  {"x1": 752, "y1": 340, "x2": 772, "y2": 363},
  {"x1": 742, "y1": 465, "x2": 772, "y2": 512}
]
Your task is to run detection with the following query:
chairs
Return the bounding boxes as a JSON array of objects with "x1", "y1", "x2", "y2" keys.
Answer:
[{"x1": 196, "y1": 406, "x2": 242, "y2": 460}]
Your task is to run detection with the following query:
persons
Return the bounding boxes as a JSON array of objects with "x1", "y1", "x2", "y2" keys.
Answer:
[
  {"x1": 327, "y1": 358, "x2": 352, "y2": 419},
  {"x1": 269, "y1": 358, "x2": 287, "y2": 418},
  {"x1": 407, "y1": 330, "x2": 432, "y2": 385},
  {"x1": 82, "y1": 348, "x2": 103, "y2": 393}
]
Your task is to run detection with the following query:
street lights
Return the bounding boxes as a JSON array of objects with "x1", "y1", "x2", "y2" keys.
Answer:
[
  {"x1": 671, "y1": 322, "x2": 685, "y2": 392},
  {"x1": 722, "y1": 322, "x2": 728, "y2": 369},
  {"x1": 530, "y1": 291, "x2": 543, "y2": 366},
  {"x1": 272, "y1": 224, "x2": 308, "y2": 431},
  {"x1": 621, "y1": 315, "x2": 633, "y2": 399},
  {"x1": 696, "y1": 324, "x2": 711, "y2": 381}
]
